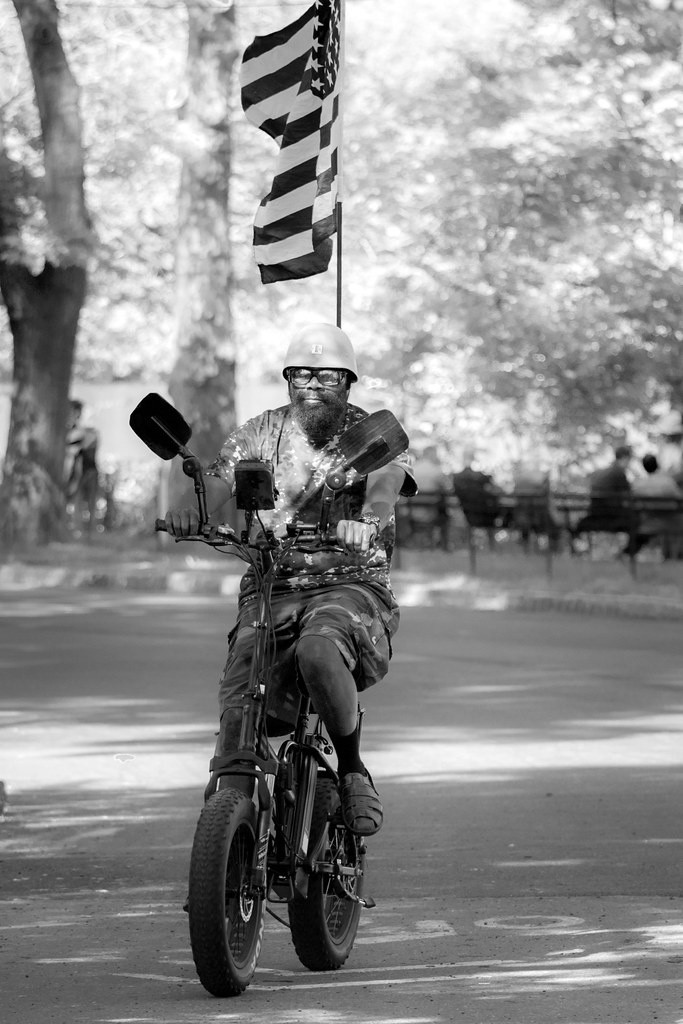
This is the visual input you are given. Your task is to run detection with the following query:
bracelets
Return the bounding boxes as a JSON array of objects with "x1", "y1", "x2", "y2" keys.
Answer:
[{"x1": 356, "y1": 512, "x2": 381, "y2": 541}]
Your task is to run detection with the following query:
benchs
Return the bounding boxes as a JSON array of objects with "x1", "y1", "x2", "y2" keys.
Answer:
[{"x1": 387, "y1": 492, "x2": 683, "y2": 578}]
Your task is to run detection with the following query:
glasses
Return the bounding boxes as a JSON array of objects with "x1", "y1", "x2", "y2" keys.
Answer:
[{"x1": 285, "y1": 366, "x2": 350, "y2": 388}]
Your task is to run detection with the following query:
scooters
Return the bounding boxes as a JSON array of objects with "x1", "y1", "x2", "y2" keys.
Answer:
[{"x1": 129, "y1": 390, "x2": 411, "y2": 1000}]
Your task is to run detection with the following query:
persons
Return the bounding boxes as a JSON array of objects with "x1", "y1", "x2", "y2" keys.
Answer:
[
  {"x1": 64, "y1": 401, "x2": 100, "y2": 492},
  {"x1": 163, "y1": 323, "x2": 420, "y2": 835},
  {"x1": 414, "y1": 436, "x2": 680, "y2": 562}
]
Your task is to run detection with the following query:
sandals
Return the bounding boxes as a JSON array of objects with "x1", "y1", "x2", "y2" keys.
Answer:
[{"x1": 340, "y1": 773, "x2": 385, "y2": 837}]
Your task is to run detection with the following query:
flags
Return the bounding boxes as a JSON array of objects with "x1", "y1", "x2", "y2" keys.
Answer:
[{"x1": 240, "y1": 0, "x2": 346, "y2": 284}]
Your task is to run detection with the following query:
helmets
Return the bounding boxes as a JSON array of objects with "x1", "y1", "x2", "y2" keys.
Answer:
[{"x1": 281, "y1": 324, "x2": 359, "y2": 382}]
